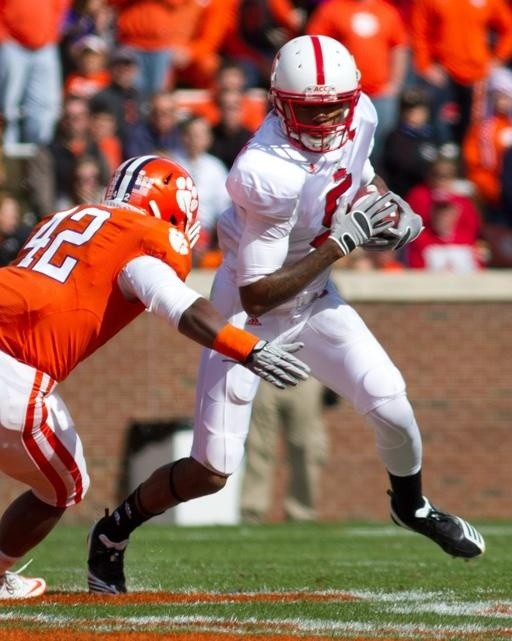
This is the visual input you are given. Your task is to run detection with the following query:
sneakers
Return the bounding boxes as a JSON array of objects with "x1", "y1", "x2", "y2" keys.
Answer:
[
  {"x1": 387, "y1": 489, "x2": 485, "y2": 563},
  {"x1": 86, "y1": 509, "x2": 130, "y2": 593},
  {"x1": 0, "y1": 559, "x2": 47, "y2": 599}
]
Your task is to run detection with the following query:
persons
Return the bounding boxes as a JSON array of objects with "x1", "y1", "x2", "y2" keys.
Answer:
[
  {"x1": 0, "y1": 153, "x2": 314, "y2": 602},
  {"x1": 236, "y1": 370, "x2": 330, "y2": 524},
  {"x1": 85, "y1": 34, "x2": 487, "y2": 595}
]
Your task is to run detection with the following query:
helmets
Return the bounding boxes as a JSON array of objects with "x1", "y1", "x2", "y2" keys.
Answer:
[
  {"x1": 102, "y1": 152, "x2": 200, "y2": 252},
  {"x1": 269, "y1": 35, "x2": 363, "y2": 156}
]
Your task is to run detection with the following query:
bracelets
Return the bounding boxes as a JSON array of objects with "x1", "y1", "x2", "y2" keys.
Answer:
[{"x1": 212, "y1": 322, "x2": 260, "y2": 363}]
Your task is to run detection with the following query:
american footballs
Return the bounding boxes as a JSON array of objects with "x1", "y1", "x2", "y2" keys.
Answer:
[{"x1": 345, "y1": 185, "x2": 400, "y2": 229}]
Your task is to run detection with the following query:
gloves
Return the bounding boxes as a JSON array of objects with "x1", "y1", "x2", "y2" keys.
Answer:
[
  {"x1": 241, "y1": 338, "x2": 312, "y2": 391},
  {"x1": 327, "y1": 191, "x2": 424, "y2": 255}
]
